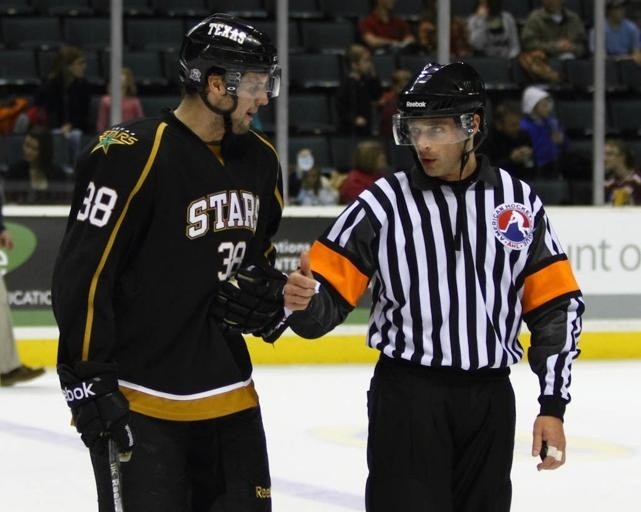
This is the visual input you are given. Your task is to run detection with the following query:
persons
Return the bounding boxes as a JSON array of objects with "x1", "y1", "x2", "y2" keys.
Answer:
[
  {"x1": 0, "y1": 1, "x2": 640, "y2": 208},
  {"x1": 50, "y1": 15, "x2": 293, "y2": 512},
  {"x1": 285, "y1": 62, "x2": 585, "y2": 512},
  {"x1": 0, "y1": 178, "x2": 46, "y2": 386}
]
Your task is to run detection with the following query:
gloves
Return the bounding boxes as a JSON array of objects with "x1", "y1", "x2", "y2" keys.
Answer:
[
  {"x1": 58, "y1": 355, "x2": 135, "y2": 456},
  {"x1": 210, "y1": 258, "x2": 290, "y2": 345}
]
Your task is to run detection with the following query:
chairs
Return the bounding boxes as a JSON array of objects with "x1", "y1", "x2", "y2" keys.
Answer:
[{"x1": 0, "y1": 1, "x2": 641, "y2": 205}]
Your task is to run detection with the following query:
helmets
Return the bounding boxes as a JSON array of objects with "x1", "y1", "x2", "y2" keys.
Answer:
[
  {"x1": 177, "y1": 13, "x2": 282, "y2": 99},
  {"x1": 391, "y1": 60, "x2": 487, "y2": 146}
]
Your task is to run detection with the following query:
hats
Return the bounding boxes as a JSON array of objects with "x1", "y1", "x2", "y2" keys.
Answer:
[{"x1": 521, "y1": 86, "x2": 548, "y2": 114}]
